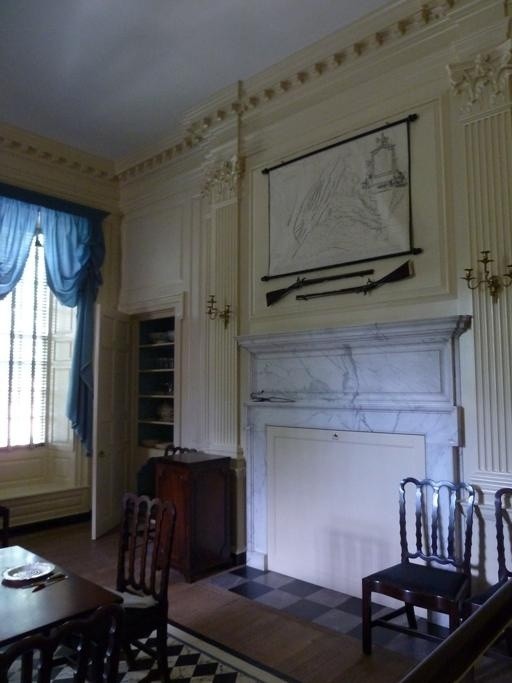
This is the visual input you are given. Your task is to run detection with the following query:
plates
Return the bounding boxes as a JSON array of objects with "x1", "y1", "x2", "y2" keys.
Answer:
[{"x1": 4, "y1": 562, "x2": 53, "y2": 584}]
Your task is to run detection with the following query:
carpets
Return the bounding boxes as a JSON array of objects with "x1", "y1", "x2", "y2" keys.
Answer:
[{"x1": 0, "y1": 617, "x2": 302, "y2": 683}]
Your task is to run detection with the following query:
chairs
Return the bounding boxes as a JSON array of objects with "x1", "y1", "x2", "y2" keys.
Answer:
[
  {"x1": 0, "y1": 602, "x2": 127, "y2": 683},
  {"x1": 361, "y1": 477, "x2": 475, "y2": 655},
  {"x1": 0, "y1": 505, "x2": 10, "y2": 549},
  {"x1": 105, "y1": 491, "x2": 176, "y2": 683},
  {"x1": 463, "y1": 487, "x2": 512, "y2": 620}
]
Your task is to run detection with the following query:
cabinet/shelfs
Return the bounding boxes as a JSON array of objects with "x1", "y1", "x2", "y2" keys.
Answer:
[
  {"x1": 153, "y1": 451, "x2": 230, "y2": 582},
  {"x1": 89, "y1": 292, "x2": 185, "y2": 540}
]
